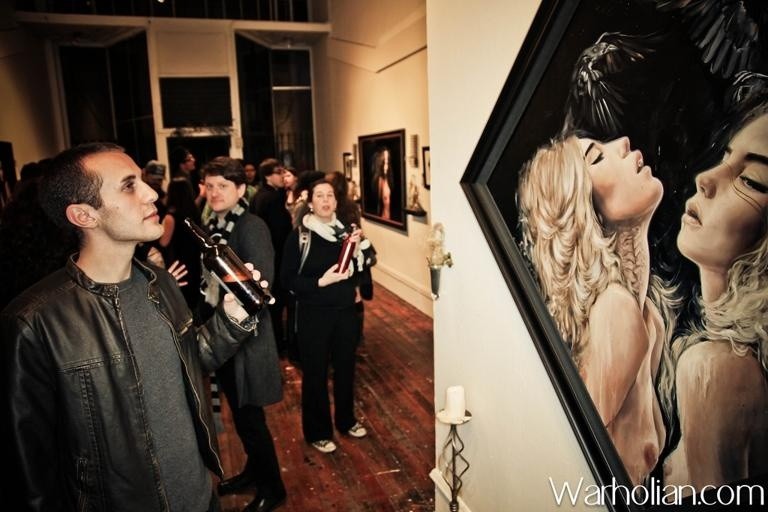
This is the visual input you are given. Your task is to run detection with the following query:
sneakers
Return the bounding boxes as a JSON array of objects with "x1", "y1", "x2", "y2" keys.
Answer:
[
  {"x1": 348, "y1": 423, "x2": 367, "y2": 438},
  {"x1": 313, "y1": 439, "x2": 336, "y2": 453}
]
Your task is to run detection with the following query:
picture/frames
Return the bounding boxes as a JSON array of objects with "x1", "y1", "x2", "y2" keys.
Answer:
[
  {"x1": 460, "y1": 0, "x2": 768, "y2": 512},
  {"x1": 343, "y1": 128, "x2": 430, "y2": 232}
]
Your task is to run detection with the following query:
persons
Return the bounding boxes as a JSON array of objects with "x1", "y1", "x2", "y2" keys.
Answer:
[
  {"x1": 513, "y1": 136, "x2": 668, "y2": 497},
  {"x1": 0, "y1": 139, "x2": 376, "y2": 510},
  {"x1": 371, "y1": 148, "x2": 394, "y2": 221},
  {"x1": 659, "y1": 101, "x2": 768, "y2": 502}
]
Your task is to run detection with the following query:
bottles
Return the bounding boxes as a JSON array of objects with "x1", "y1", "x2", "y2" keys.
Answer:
[
  {"x1": 182, "y1": 213, "x2": 271, "y2": 317},
  {"x1": 335, "y1": 225, "x2": 359, "y2": 276}
]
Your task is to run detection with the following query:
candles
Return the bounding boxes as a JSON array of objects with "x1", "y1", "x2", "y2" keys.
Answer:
[{"x1": 444, "y1": 384, "x2": 466, "y2": 422}]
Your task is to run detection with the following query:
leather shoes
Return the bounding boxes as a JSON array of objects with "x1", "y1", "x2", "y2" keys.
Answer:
[
  {"x1": 243, "y1": 494, "x2": 286, "y2": 512},
  {"x1": 218, "y1": 474, "x2": 250, "y2": 493}
]
